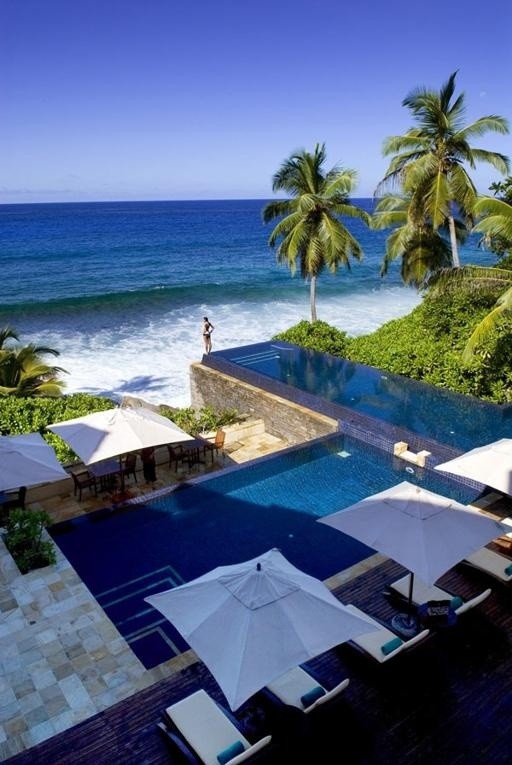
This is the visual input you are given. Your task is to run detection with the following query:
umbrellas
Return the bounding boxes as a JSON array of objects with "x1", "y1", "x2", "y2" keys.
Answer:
[
  {"x1": 433, "y1": 436, "x2": 511, "y2": 499},
  {"x1": 143, "y1": 547, "x2": 382, "y2": 713},
  {"x1": 1, "y1": 432, "x2": 74, "y2": 506},
  {"x1": 45, "y1": 403, "x2": 197, "y2": 488},
  {"x1": 316, "y1": 481, "x2": 511, "y2": 623}
]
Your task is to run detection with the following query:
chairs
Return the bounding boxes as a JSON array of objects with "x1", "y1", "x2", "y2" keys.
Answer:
[
  {"x1": 70, "y1": 455, "x2": 137, "y2": 501},
  {"x1": 167, "y1": 430, "x2": 225, "y2": 473}
]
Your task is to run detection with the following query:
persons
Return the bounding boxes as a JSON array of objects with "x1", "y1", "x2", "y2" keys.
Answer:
[
  {"x1": 140, "y1": 447, "x2": 157, "y2": 492},
  {"x1": 202, "y1": 316, "x2": 214, "y2": 354}
]
